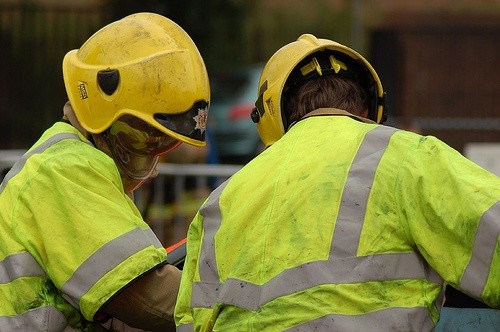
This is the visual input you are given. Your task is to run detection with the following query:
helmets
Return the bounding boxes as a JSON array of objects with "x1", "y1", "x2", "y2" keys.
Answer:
[
  {"x1": 251, "y1": 34, "x2": 388, "y2": 149},
  {"x1": 62, "y1": 13, "x2": 211, "y2": 147}
]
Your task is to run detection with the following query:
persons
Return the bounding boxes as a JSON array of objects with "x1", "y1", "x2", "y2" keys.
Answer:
[
  {"x1": 82, "y1": 0, "x2": 411, "y2": 219},
  {"x1": 0, "y1": 13, "x2": 210, "y2": 332},
  {"x1": 174, "y1": 34, "x2": 500, "y2": 332}
]
